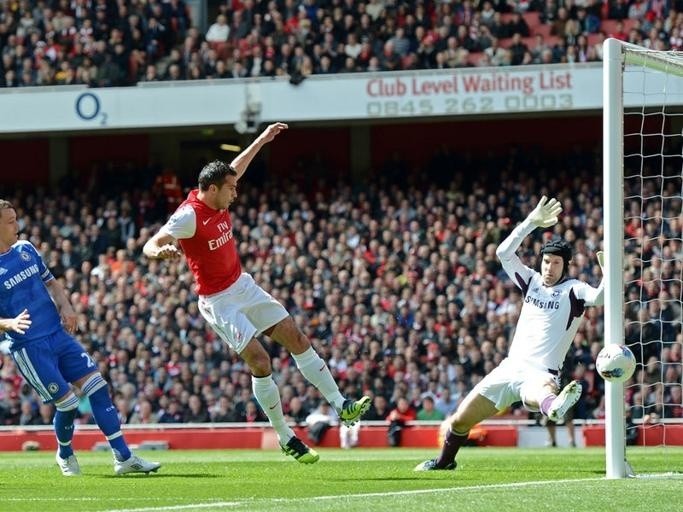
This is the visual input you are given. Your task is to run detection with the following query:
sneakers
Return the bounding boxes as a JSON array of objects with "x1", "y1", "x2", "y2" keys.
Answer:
[
  {"x1": 55, "y1": 449, "x2": 80, "y2": 476},
  {"x1": 114, "y1": 455, "x2": 160, "y2": 473},
  {"x1": 340, "y1": 396, "x2": 372, "y2": 428},
  {"x1": 415, "y1": 458, "x2": 457, "y2": 471},
  {"x1": 548, "y1": 380, "x2": 583, "y2": 422},
  {"x1": 280, "y1": 436, "x2": 319, "y2": 463}
]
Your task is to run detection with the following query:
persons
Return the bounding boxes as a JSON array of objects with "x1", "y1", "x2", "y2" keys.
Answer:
[
  {"x1": 0, "y1": 1, "x2": 682, "y2": 85},
  {"x1": 141, "y1": 121, "x2": 376, "y2": 466},
  {"x1": 0, "y1": 171, "x2": 683, "y2": 422},
  {"x1": 414, "y1": 193, "x2": 606, "y2": 472},
  {"x1": 0, "y1": 198, "x2": 164, "y2": 476}
]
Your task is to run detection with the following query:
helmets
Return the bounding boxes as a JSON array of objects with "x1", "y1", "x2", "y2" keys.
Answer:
[{"x1": 540, "y1": 240, "x2": 572, "y2": 260}]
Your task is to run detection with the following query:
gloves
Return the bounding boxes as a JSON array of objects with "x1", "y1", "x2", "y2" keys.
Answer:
[{"x1": 528, "y1": 195, "x2": 563, "y2": 228}]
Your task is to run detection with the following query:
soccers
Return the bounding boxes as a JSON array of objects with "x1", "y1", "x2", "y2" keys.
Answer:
[{"x1": 595, "y1": 344, "x2": 636, "y2": 384}]
